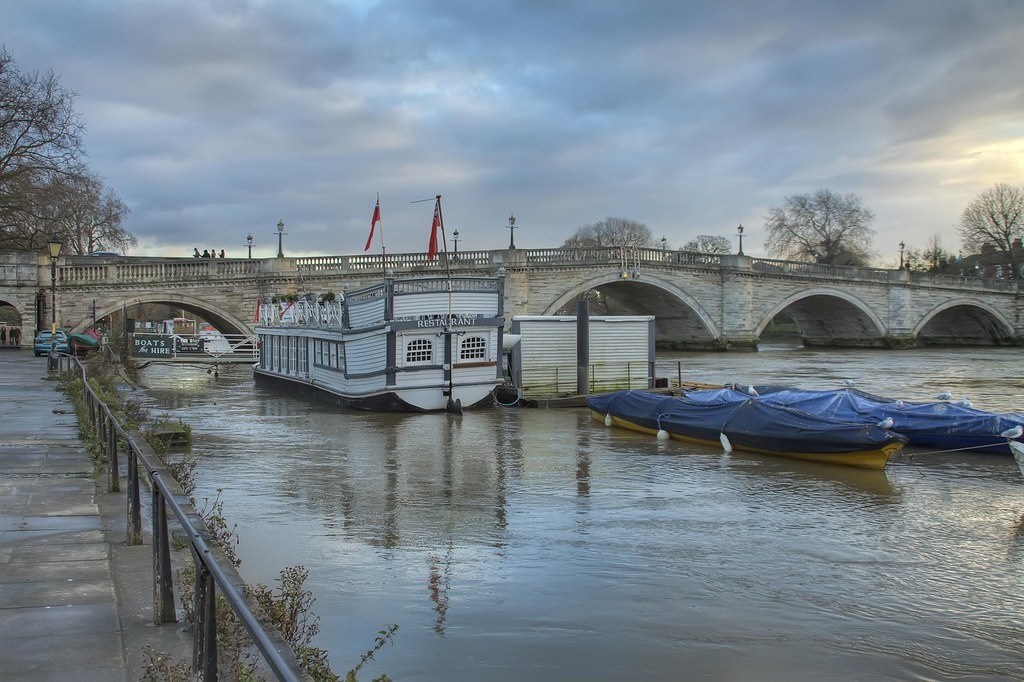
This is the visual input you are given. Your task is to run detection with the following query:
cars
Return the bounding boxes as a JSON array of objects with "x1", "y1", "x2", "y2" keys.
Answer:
[
  {"x1": 86, "y1": 251, "x2": 121, "y2": 257},
  {"x1": 34, "y1": 330, "x2": 70, "y2": 357}
]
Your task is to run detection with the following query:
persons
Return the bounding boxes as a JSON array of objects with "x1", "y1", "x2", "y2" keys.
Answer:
[
  {"x1": 193, "y1": 248, "x2": 199, "y2": 257},
  {"x1": 211, "y1": 249, "x2": 215, "y2": 258},
  {"x1": 199, "y1": 250, "x2": 210, "y2": 258},
  {"x1": 218, "y1": 249, "x2": 224, "y2": 258},
  {"x1": 9, "y1": 326, "x2": 21, "y2": 345},
  {"x1": 0, "y1": 327, "x2": 7, "y2": 344}
]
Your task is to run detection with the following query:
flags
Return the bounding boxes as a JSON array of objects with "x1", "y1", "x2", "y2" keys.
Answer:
[
  {"x1": 365, "y1": 198, "x2": 380, "y2": 251},
  {"x1": 428, "y1": 201, "x2": 440, "y2": 262}
]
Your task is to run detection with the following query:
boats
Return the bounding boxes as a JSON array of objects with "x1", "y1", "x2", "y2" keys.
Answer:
[
  {"x1": 586, "y1": 390, "x2": 912, "y2": 471},
  {"x1": 842, "y1": 385, "x2": 1024, "y2": 453},
  {"x1": 252, "y1": 195, "x2": 510, "y2": 419}
]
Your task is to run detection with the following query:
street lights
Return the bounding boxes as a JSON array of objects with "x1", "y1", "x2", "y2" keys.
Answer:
[
  {"x1": 738, "y1": 223, "x2": 746, "y2": 257},
  {"x1": 898, "y1": 240, "x2": 906, "y2": 270},
  {"x1": 506, "y1": 213, "x2": 519, "y2": 249},
  {"x1": 452, "y1": 229, "x2": 461, "y2": 253},
  {"x1": 48, "y1": 233, "x2": 63, "y2": 377},
  {"x1": 272, "y1": 219, "x2": 288, "y2": 259},
  {"x1": 661, "y1": 235, "x2": 667, "y2": 250},
  {"x1": 243, "y1": 234, "x2": 257, "y2": 260}
]
